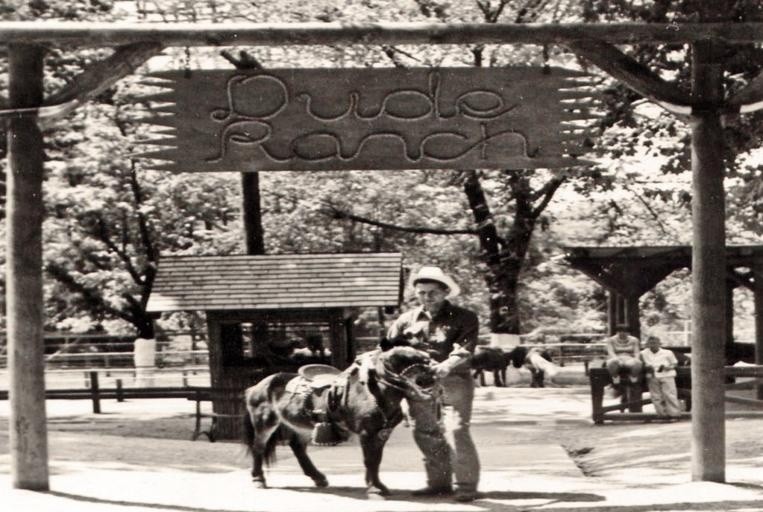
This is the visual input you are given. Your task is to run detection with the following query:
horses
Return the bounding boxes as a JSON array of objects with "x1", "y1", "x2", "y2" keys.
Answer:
[{"x1": 240, "y1": 334, "x2": 440, "y2": 501}]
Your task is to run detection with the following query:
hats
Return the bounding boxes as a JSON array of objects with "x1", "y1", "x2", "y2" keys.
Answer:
[{"x1": 410, "y1": 266, "x2": 461, "y2": 301}]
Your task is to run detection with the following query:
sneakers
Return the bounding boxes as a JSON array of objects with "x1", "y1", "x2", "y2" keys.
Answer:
[
  {"x1": 612, "y1": 377, "x2": 642, "y2": 393},
  {"x1": 650, "y1": 413, "x2": 680, "y2": 423},
  {"x1": 410, "y1": 486, "x2": 455, "y2": 498},
  {"x1": 451, "y1": 488, "x2": 476, "y2": 502}
]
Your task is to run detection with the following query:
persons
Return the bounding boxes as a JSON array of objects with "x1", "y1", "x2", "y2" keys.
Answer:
[
  {"x1": 604, "y1": 324, "x2": 643, "y2": 391},
  {"x1": 352, "y1": 266, "x2": 480, "y2": 503},
  {"x1": 525, "y1": 341, "x2": 552, "y2": 388},
  {"x1": 638, "y1": 336, "x2": 680, "y2": 422}
]
